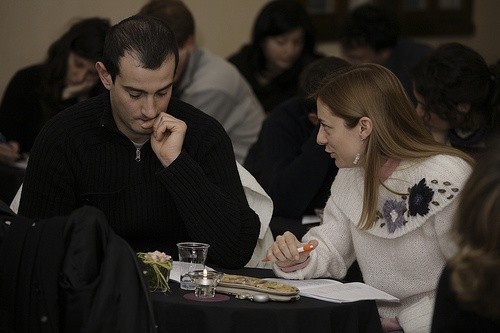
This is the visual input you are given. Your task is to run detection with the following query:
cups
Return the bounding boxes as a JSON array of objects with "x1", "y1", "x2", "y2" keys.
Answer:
[
  {"x1": 176, "y1": 242, "x2": 210, "y2": 291},
  {"x1": 188, "y1": 271, "x2": 226, "y2": 298}
]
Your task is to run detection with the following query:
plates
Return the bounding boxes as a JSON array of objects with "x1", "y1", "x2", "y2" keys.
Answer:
[
  {"x1": 213, "y1": 286, "x2": 299, "y2": 301},
  {"x1": 217, "y1": 274, "x2": 300, "y2": 295}
]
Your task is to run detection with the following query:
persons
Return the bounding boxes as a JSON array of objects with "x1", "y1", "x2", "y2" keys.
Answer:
[
  {"x1": 139, "y1": 0, "x2": 270, "y2": 165},
  {"x1": 411, "y1": 41, "x2": 500, "y2": 178},
  {"x1": 222, "y1": 0, "x2": 330, "y2": 118},
  {"x1": 15, "y1": 14, "x2": 261, "y2": 271},
  {"x1": 432, "y1": 140, "x2": 500, "y2": 333},
  {"x1": 242, "y1": 5, "x2": 402, "y2": 244},
  {"x1": 267, "y1": 63, "x2": 478, "y2": 333},
  {"x1": 0, "y1": 17, "x2": 112, "y2": 156}
]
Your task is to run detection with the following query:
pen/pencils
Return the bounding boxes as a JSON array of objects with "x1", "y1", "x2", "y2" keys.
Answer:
[{"x1": 262, "y1": 244, "x2": 315, "y2": 262}]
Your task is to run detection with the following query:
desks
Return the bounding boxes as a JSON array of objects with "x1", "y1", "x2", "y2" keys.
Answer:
[{"x1": 149, "y1": 267, "x2": 384, "y2": 333}]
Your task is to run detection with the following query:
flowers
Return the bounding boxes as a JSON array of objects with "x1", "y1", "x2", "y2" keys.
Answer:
[{"x1": 139, "y1": 250, "x2": 173, "y2": 296}]
